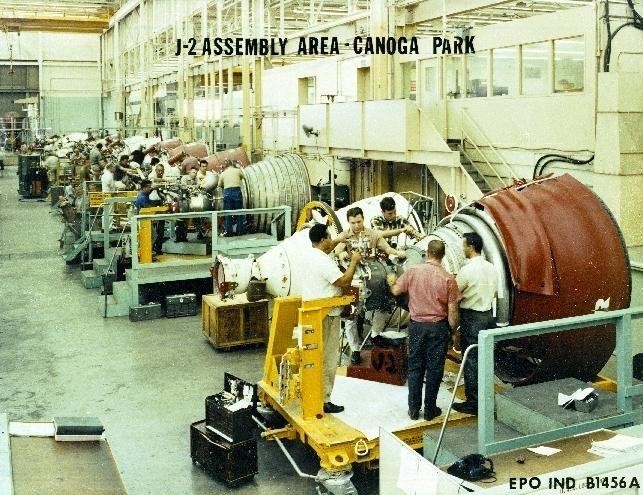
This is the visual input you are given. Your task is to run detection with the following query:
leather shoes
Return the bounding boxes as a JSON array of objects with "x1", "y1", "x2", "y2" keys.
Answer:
[
  {"x1": 452, "y1": 401, "x2": 480, "y2": 417},
  {"x1": 324, "y1": 402, "x2": 344, "y2": 413},
  {"x1": 407, "y1": 410, "x2": 420, "y2": 420},
  {"x1": 424, "y1": 408, "x2": 441, "y2": 422},
  {"x1": 350, "y1": 351, "x2": 360, "y2": 363}
]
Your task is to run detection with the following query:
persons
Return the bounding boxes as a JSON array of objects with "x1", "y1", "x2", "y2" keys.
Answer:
[
  {"x1": 102, "y1": 162, "x2": 115, "y2": 233},
  {"x1": 386, "y1": 240, "x2": 461, "y2": 420},
  {"x1": 130, "y1": 144, "x2": 147, "y2": 163},
  {"x1": 114, "y1": 155, "x2": 137, "y2": 189},
  {"x1": 336, "y1": 207, "x2": 407, "y2": 365},
  {"x1": 134, "y1": 180, "x2": 157, "y2": 262},
  {"x1": 220, "y1": 159, "x2": 244, "y2": 237},
  {"x1": 451, "y1": 231, "x2": 499, "y2": 417},
  {"x1": 195, "y1": 160, "x2": 212, "y2": 239},
  {"x1": 298, "y1": 224, "x2": 361, "y2": 415},
  {"x1": 20, "y1": 135, "x2": 103, "y2": 208},
  {"x1": 370, "y1": 197, "x2": 426, "y2": 250},
  {"x1": 149, "y1": 163, "x2": 168, "y2": 256},
  {"x1": 173, "y1": 167, "x2": 197, "y2": 243}
]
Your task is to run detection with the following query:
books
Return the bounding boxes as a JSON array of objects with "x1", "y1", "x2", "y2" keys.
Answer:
[
  {"x1": 587, "y1": 435, "x2": 643, "y2": 456},
  {"x1": 51, "y1": 416, "x2": 104, "y2": 441}
]
